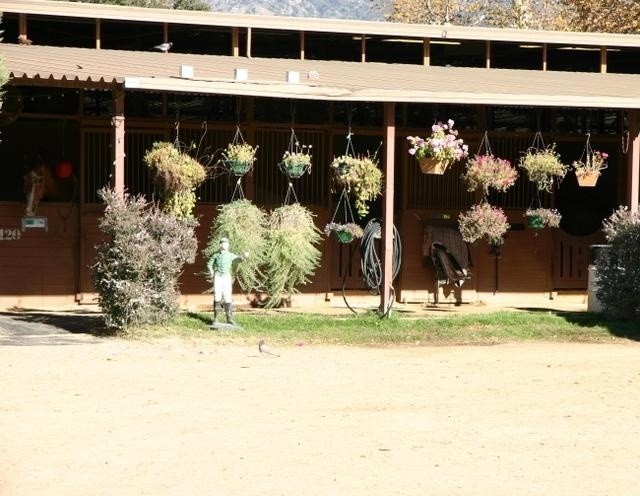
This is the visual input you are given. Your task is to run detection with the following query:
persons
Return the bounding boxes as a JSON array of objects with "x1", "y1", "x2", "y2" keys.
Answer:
[{"x1": 208, "y1": 238, "x2": 249, "y2": 325}]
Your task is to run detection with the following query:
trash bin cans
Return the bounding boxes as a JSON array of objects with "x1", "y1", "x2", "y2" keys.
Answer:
[{"x1": 586, "y1": 242, "x2": 628, "y2": 320}]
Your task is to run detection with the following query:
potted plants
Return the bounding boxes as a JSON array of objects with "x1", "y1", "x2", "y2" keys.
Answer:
[
  {"x1": 522, "y1": 209, "x2": 561, "y2": 228},
  {"x1": 520, "y1": 143, "x2": 566, "y2": 192},
  {"x1": 278, "y1": 136, "x2": 313, "y2": 178},
  {"x1": 326, "y1": 190, "x2": 365, "y2": 243},
  {"x1": 270, "y1": 182, "x2": 324, "y2": 251},
  {"x1": 144, "y1": 138, "x2": 208, "y2": 222},
  {"x1": 207, "y1": 179, "x2": 270, "y2": 250},
  {"x1": 222, "y1": 126, "x2": 257, "y2": 176},
  {"x1": 333, "y1": 132, "x2": 383, "y2": 217}
]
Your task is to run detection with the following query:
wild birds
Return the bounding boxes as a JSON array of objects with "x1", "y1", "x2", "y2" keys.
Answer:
[
  {"x1": 17, "y1": 34, "x2": 32, "y2": 45},
  {"x1": 152, "y1": 42, "x2": 173, "y2": 53},
  {"x1": 259, "y1": 340, "x2": 281, "y2": 360}
]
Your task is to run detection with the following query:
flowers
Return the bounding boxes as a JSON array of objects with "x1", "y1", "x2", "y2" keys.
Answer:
[
  {"x1": 462, "y1": 152, "x2": 519, "y2": 194},
  {"x1": 456, "y1": 202, "x2": 511, "y2": 246},
  {"x1": 573, "y1": 150, "x2": 609, "y2": 177},
  {"x1": 406, "y1": 119, "x2": 469, "y2": 167}
]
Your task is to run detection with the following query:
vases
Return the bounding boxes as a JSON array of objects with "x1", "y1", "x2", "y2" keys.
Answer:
[
  {"x1": 418, "y1": 156, "x2": 449, "y2": 176},
  {"x1": 577, "y1": 172, "x2": 598, "y2": 187}
]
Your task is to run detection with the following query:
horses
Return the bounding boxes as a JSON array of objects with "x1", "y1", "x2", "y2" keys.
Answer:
[{"x1": 24, "y1": 164, "x2": 62, "y2": 217}]
